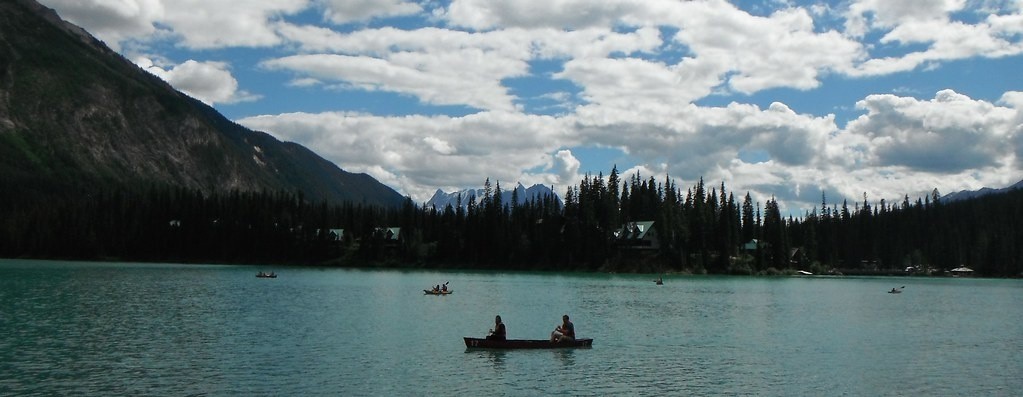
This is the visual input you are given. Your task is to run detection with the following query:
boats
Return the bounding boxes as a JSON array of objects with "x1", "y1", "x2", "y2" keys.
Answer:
[
  {"x1": 423, "y1": 288, "x2": 454, "y2": 295},
  {"x1": 462, "y1": 336, "x2": 594, "y2": 353}
]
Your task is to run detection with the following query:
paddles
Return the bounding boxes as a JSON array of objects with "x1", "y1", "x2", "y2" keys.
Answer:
[
  {"x1": 445, "y1": 282, "x2": 449, "y2": 286},
  {"x1": 551, "y1": 326, "x2": 559, "y2": 337}
]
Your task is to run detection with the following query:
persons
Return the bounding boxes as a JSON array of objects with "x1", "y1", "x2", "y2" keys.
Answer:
[
  {"x1": 658, "y1": 275, "x2": 662, "y2": 283},
  {"x1": 441, "y1": 284, "x2": 447, "y2": 291},
  {"x1": 892, "y1": 288, "x2": 895, "y2": 292},
  {"x1": 485, "y1": 315, "x2": 506, "y2": 341},
  {"x1": 434, "y1": 285, "x2": 439, "y2": 291},
  {"x1": 550, "y1": 315, "x2": 575, "y2": 342},
  {"x1": 258, "y1": 269, "x2": 274, "y2": 277}
]
